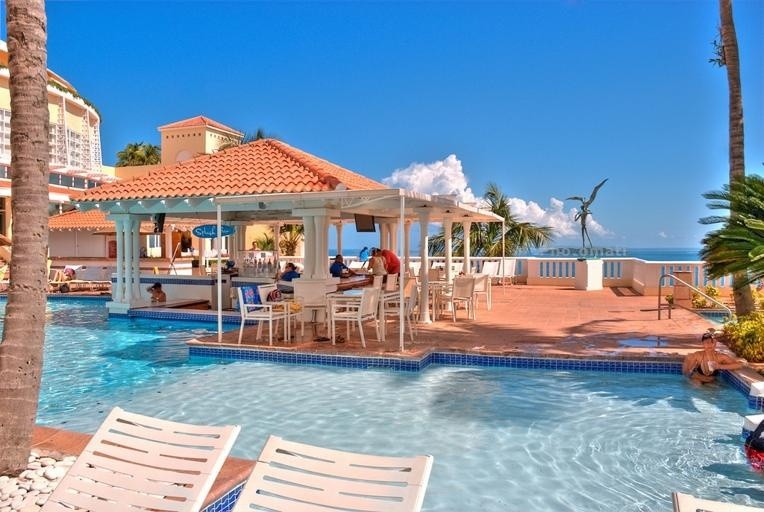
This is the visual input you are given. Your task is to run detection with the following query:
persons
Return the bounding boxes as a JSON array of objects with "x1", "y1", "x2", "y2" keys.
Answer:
[
  {"x1": 50, "y1": 270, "x2": 72, "y2": 282},
  {"x1": 146, "y1": 282, "x2": 166, "y2": 303},
  {"x1": 330, "y1": 247, "x2": 400, "y2": 283},
  {"x1": 249, "y1": 241, "x2": 261, "y2": 251},
  {"x1": 277, "y1": 263, "x2": 300, "y2": 293},
  {"x1": 682, "y1": 333, "x2": 738, "y2": 387}
]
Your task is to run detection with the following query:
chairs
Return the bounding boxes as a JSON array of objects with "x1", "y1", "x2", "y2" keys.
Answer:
[
  {"x1": 35, "y1": 403, "x2": 242, "y2": 511},
  {"x1": 225, "y1": 432, "x2": 435, "y2": 512},
  {"x1": 233, "y1": 265, "x2": 492, "y2": 353},
  {"x1": 669, "y1": 489, "x2": 763, "y2": 511}
]
